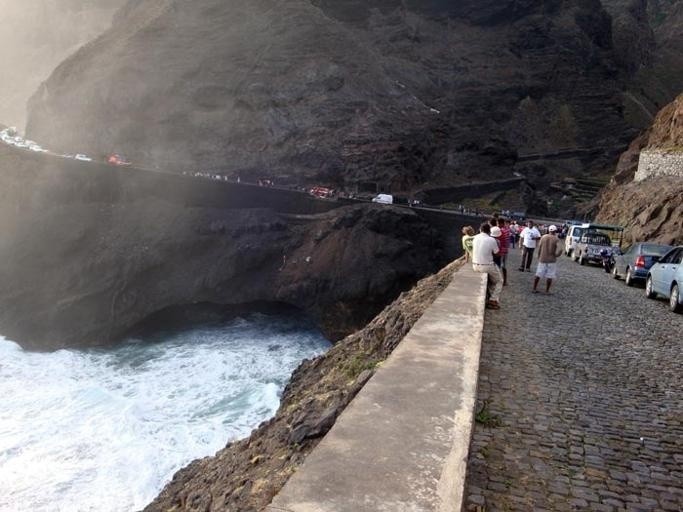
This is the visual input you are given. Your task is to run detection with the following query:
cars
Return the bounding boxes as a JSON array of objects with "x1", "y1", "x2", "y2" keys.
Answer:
[
  {"x1": 0, "y1": 127, "x2": 133, "y2": 168},
  {"x1": 564, "y1": 224, "x2": 683, "y2": 312}
]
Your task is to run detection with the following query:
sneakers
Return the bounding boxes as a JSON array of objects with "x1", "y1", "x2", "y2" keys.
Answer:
[{"x1": 532, "y1": 290, "x2": 553, "y2": 295}]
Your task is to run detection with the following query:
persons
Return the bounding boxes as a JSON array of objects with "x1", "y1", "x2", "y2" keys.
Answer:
[{"x1": 461, "y1": 212, "x2": 569, "y2": 309}]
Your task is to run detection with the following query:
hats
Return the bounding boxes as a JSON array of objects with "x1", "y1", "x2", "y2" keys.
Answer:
[
  {"x1": 549, "y1": 225, "x2": 557, "y2": 231},
  {"x1": 490, "y1": 227, "x2": 502, "y2": 237}
]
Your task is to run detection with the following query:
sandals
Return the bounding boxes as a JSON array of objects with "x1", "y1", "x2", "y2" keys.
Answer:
[{"x1": 487, "y1": 303, "x2": 500, "y2": 309}]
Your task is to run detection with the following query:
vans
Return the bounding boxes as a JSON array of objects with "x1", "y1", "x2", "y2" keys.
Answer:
[{"x1": 371, "y1": 193, "x2": 393, "y2": 204}]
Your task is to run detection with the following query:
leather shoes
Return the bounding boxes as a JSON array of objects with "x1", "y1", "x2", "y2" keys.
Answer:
[{"x1": 518, "y1": 268, "x2": 529, "y2": 271}]
